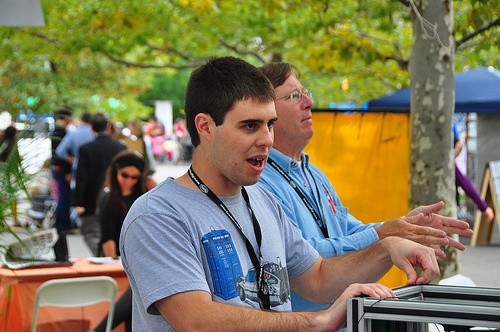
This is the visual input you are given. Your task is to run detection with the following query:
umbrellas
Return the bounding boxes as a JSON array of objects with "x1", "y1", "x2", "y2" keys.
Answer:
[{"x1": 364, "y1": 64, "x2": 500, "y2": 220}]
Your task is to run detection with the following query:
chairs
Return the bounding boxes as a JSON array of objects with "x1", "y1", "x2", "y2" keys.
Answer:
[{"x1": 31, "y1": 275, "x2": 120, "y2": 331}]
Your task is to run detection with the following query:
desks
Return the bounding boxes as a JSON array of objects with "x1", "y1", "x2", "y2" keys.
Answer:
[{"x1": 0, "y1": 256, "x2": 132, "y2": 332}]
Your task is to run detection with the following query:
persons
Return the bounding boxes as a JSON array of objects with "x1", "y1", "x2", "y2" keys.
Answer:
[
  {"x1": 119, "y1": 57, "x2": 442, "y2": 332},
  {"x1": 110, "y1": 113, "x2": 193, "y2": 190},
  {"x1": 454, "y1": 162, "x2": 494, "y2": 221},
  {"x1": 256, "y1": 61, "x2": 475, "y2": 332},
  {"x1": 0, "y1": 102, "x2": 95, "y2": 263},
  {"x1": 94, "y1": 150, "x2": 151, "y2": 259},
  {"x1": 74, "y1": 113, "x2": 129, "y2": 259},
  {"x1": 451, "y1": 123, "x2": 465, "y2": 160}
]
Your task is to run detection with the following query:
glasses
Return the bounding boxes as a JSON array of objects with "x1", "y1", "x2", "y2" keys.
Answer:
[
  {"x1": 274, "y1": 87, "x2": 313, "y2": 104},
  {"x1": 121, "y1": 171, "x2": 141, "y2": 179}
]
